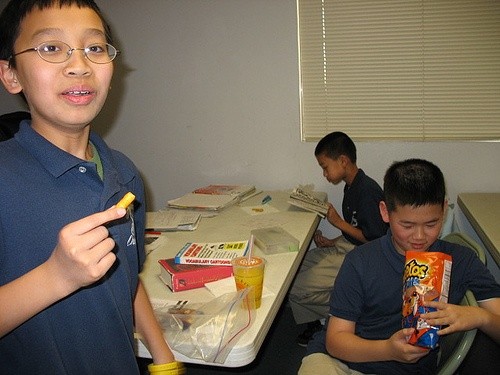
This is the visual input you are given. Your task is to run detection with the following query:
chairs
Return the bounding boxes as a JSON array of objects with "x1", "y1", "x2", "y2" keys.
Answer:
[
  {"x1": 443, "y1": 232, "x2": 487, "y2": 266},
  {"x1": 436, "y1": 289, "x2": 479, "y2": 375}
]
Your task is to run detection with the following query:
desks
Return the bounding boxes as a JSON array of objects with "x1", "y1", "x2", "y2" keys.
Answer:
[
  {"x1": 132, "y1": 190, "x2": 327, "y2": 368},
  {"x1": 457, "y1": 193, "x2": 500, "y2": 267}
]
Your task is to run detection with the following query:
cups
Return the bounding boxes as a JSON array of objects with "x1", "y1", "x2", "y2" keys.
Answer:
[{"x1": 231, "y1": 257, "x2": 264, "y2": 310}]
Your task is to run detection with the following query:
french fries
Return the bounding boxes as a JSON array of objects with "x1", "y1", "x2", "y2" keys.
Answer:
[{"x1": 115, "y1": 192, "x2": 136, "y2": 209}]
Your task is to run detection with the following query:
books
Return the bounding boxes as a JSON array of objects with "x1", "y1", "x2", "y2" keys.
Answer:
[
  {"x1": 145, "y1": 184, "x2": 262, "y2": 231},
  {"x1": 156, "y1": 258, "x2": 234, "y2": 293},
  {"x1": 288, "y1": 185, "x2": 329, "y2": 219},
  {"x1": 175, "y1": 240, "x2": 250, "y2": 266}
]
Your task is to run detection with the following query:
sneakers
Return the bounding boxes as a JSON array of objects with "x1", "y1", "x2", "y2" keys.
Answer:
[{"x1": 295, "y1": 319, "x2": 326, "y2": 347}]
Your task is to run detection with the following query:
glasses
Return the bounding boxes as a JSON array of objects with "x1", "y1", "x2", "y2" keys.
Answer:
[{"x1": 7, "y1": 40, "x2": 121, "y2": 64}]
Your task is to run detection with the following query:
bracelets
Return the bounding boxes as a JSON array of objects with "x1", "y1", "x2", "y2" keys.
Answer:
[{"x1": 148, "y1": 362, "x2": 186, "y2": 375}]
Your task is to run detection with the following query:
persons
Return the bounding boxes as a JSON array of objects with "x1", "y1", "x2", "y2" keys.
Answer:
[
  {"x1": 298, "y1": 158, "x2": 500, "y2": 375},
  {"x1": 289, "y1": 131, "x2": 390, "y2": 347},
  {"x1": 0, "y1": 0, "x2": 178, "y2": 375}
]
